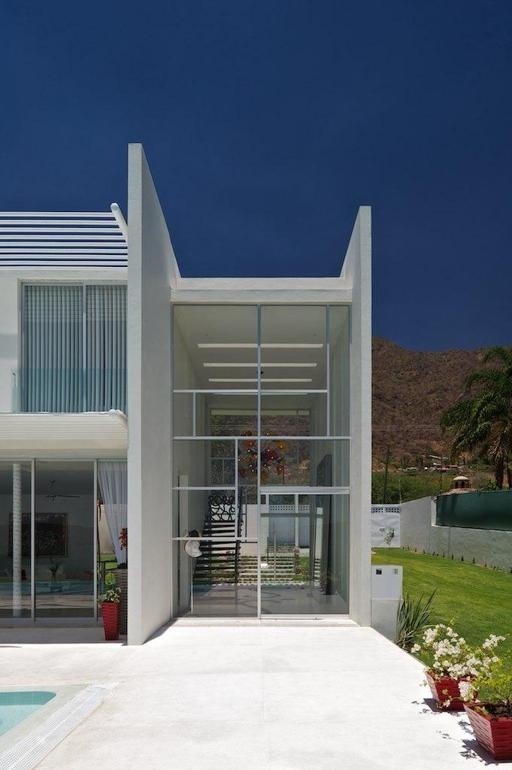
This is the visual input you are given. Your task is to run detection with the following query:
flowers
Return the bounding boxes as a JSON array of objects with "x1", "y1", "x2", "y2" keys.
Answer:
[
  {"x1": 407, "y1": 612, "x2": 512, "y2": 719},
  {"x1": 96, "y1": 586, "x2": 122, "y2": 608}
]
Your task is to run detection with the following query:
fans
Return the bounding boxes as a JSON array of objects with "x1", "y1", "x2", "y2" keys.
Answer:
[{"x1": 42, "y1": 480, "x2": 81, "y2": 498}]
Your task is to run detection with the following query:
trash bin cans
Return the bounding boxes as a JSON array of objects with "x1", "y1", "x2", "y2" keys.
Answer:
[{"x1": 371, "y1": 564, "x2": 404, "y2": 643}]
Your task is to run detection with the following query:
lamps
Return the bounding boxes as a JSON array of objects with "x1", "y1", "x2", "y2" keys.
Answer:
[{"x1": 234, "y1": 372, "x2": 286, "y2": 485}]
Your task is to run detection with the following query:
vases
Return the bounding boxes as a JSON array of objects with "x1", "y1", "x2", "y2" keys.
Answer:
[
  {"x1": 101, "y1": 601, "x2": 120, "y2": 640},
  {"x1": 462, "y1": 703, "x2": 512, "y2": 760}
]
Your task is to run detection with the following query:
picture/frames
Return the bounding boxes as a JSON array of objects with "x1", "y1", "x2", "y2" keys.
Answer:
[{"x1": 8, "y1": 512, "x2": 69, "y2": 558}]
